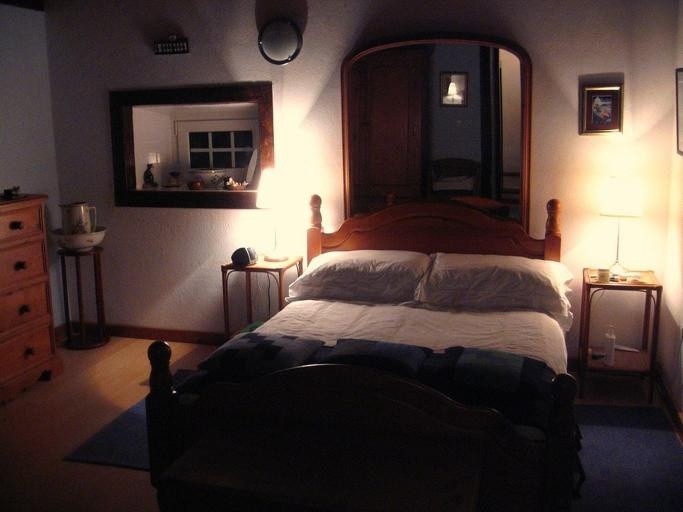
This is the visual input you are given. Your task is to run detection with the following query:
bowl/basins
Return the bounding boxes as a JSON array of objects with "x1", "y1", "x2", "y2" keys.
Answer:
[
  {"x1": 184, "y1": 167, "x2": 226, "y2": 190},
  {"x1": 52, "y1": 226, "x2": 107, "y2": 252}
]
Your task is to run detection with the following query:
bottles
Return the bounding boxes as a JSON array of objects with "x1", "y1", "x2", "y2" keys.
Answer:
[
  {"x1": 605, "y1": 325, "x2": 616, "y2": 367},
  {"x1": 167, "y1": 172, "x2": 181, "y2": 187},
  {"x1": 598, "y1": 268, "x2": 628, "y2": 284}
]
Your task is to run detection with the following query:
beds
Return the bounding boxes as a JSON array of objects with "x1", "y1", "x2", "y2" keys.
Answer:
[{"x1": 144, "y1": 196, "x2": 571, "y2": 511}]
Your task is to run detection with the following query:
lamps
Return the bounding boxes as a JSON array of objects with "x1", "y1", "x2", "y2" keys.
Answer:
[
  {"x1": 598, "y1": 170, "x2": 648, "y2": 278},
  {"x1": 256, "y1": 165, "x2": 297, "y2": 263}
]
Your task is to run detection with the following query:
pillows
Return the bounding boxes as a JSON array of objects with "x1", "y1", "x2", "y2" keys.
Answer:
[
  {"x1": 413, "y1": 251, "x2": 572, "y2": 333},
  {"x1": 285, "y1": 249, "x2": 433, "y2": 305}
]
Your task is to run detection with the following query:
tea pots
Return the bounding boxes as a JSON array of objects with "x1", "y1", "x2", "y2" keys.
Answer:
[{"x1": 58, "y1": 202, "x2": 96, "y2": 234}]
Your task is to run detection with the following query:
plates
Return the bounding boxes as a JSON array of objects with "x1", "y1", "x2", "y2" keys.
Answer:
[{"x1": 246, "y1": 148, "x2": 257, "y2": 184}]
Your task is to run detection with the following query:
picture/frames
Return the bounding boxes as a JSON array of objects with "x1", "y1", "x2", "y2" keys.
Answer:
[
  {"x1": 576, "y1": 73, "x2": 625, "y2": 135},
  {"x1": 676, "y1": 68, "x2": 683, "y2": 156},
  {"x1": 439, "y1": 70, "x2": 469, "y2": 107}
]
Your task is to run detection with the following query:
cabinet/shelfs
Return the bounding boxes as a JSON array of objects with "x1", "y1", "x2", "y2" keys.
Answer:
[
  {"x1": 57, "y1": 246, "x2": 108, "y2": 350},
  {"x1": 0, "y1": 191, "x2": 56, "y2": 403},
  {"x1": 350, "y1": 51, "x2": 429, "y2": 215}
]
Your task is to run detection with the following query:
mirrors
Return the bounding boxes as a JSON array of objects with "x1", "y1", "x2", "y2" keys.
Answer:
[{"x1": 107, "y1": 80, "x2": 275, "y2": 210}]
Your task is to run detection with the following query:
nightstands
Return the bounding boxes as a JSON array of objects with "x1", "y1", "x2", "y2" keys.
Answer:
[
  {"x1": 573, "y1": 268, "x2": 663, "y2": 410},
  {"x1": 220, "y1": 259, "x2": 304, "y2": 344}
]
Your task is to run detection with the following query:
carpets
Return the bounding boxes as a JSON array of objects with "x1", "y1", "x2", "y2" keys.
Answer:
[
  {"x1": 573, "y1": 406, "x2": 682, "y2": 512},
  {"x1": 63, "y1": 396, "x2": 150, "y2": 472}
]
